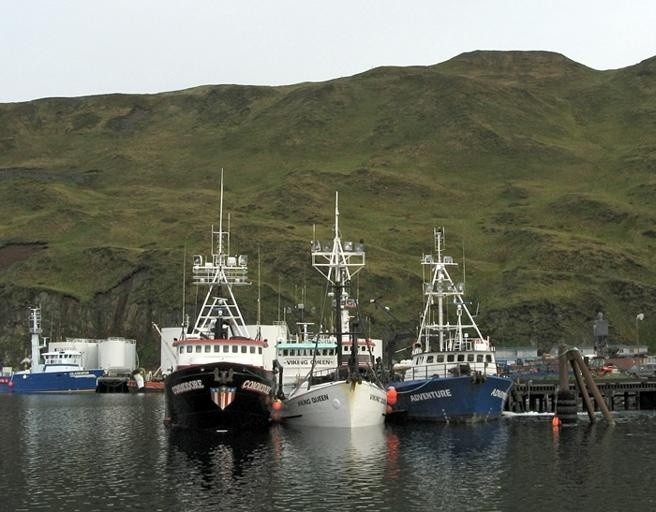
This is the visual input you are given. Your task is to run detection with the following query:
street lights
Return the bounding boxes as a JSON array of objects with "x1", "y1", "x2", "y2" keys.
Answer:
[{"x1": 635, "y1": 311, "x2": 644, "y2": 380}]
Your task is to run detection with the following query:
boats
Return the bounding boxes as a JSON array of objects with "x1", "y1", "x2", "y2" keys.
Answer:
[
  {"x1": 163, "y1": 165, "x2": 278, "y2": 435},
  {"x1": 386, "y1": 224, "x2": 516, "y2": 426},
  {"x1": 275, "y1": 191, "x2": 389, "y2": 430},
  {"x1": 0, "y1": 302, "x2": 106, "y2": 396}
]
